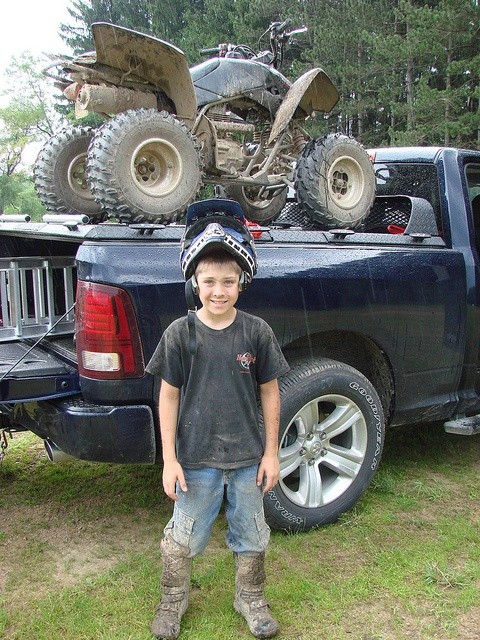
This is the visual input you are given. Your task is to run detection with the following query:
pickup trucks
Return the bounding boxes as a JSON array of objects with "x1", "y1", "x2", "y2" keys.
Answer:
[{"x1": 0, "y1": 146, "x2": 480, "y2": 534}]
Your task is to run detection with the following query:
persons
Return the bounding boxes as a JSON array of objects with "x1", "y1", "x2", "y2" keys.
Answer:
[{"x1": 144, "y1": 198, "x2": 291, "y2": 638}]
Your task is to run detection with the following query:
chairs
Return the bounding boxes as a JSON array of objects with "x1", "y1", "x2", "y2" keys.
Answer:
[{"x1": 472, "y1": 195, "x2": 480, "y2": 247}]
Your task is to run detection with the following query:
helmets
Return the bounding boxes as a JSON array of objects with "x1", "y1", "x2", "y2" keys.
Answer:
[{"x1": 180, "y1": 199, "x2": 257, "y2": 295}]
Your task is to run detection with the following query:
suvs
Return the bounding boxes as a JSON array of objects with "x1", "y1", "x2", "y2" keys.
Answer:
[{"x1": 33, "y1": 17, "x2": 378, "y2": 233}]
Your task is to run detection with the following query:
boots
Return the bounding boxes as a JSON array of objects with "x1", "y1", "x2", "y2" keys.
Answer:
[
  {"x1": 233, "y1": 550, "x2": 280, "y2": 638},
  {"x1": 151, "y1": 539, "x2": 191, "y2": 639}
]
